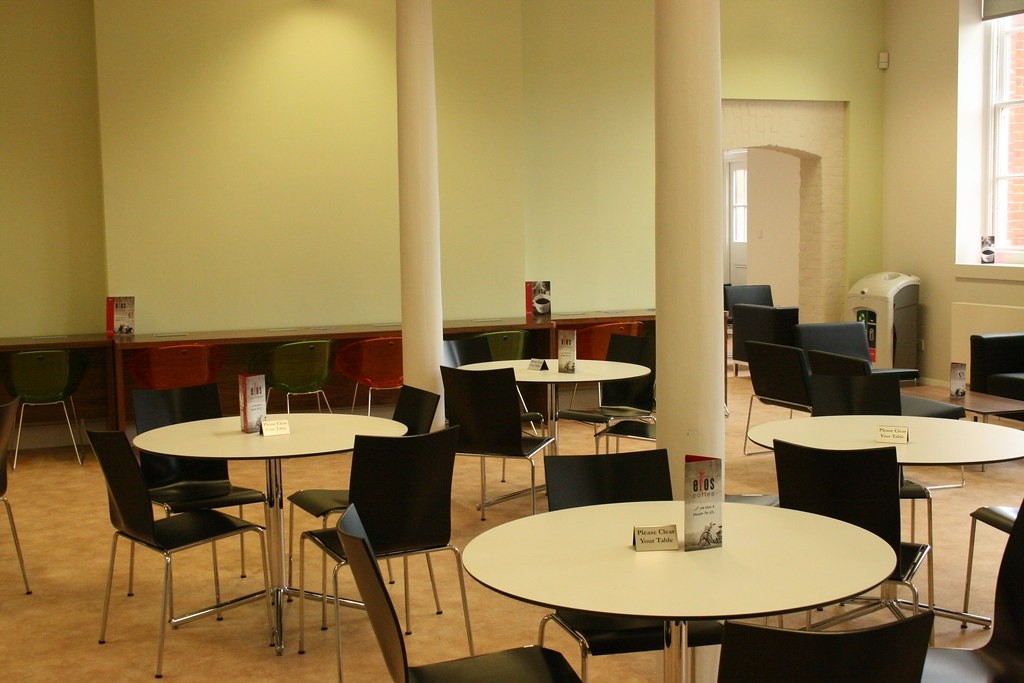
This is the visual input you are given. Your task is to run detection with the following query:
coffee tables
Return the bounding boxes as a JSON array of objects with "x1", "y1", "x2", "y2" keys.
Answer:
[{"x1": 897, "y1": 386, "x2": 1024, "y2": 472}]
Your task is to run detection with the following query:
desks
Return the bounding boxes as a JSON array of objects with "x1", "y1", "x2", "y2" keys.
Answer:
[
  {"x1": 746, "y1": 414, "x2": 1024, "y2": 632},
  {"x1": 461, "y1": 500, "x2": 898, "y2": 682},
  {"x1": 0, "y1": 308, "x2": 730, "y2": 435},
  {"x1": 131, "y1": 412, "x2": 408, "y2": 657},
  {"x1": 456, "y1": 358, "x2": 652, "y2": 510}
]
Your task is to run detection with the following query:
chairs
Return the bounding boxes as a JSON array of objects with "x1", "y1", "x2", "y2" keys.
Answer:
[
  {"x1": 536, "y1": 449, "x2": 726, "y2": 682},
  {"x1": 560, "y1": 321, "x2": 643, "y2": 410},
  {"x1": 743, "y1": 339, "x2": 815, "y2": 453},
  {"x1": 593, "y1": 420, "x2": 657, "y2": 455},
  {"x1": 808, "y1": 349, "x2": 967, "y2": 492},
  {"x1": 807, "y1": 373, "x2": 935, "y2": 607},
  {"x1": 961, "y1": 503, "x2": 1024, "y2": 629},
  {"x1": 79, "y1": 418, "x2": 276, "y2": 679},
  {"x1": 286, "y1": 384, "x2": 443, "y2": 630},
  {"x1": 463, "y1": 330, "x2": 540, "y2": 437},
  {"x1": 444, "y1": 336, "x2": 547, "y2": 482},
  {"x1": 336, "y1": 504, "x2": 583, "y2": 682},
  {"x1": 126, "y1": 381, "x2": 283, "y2": 631},
  {"x1": 10, "y1": 349, "x2": 88, "y2": 470},
  {"x1": 247, "y1": 338, "x2": 334, "y2": 415},
  {"x1": 126, "y1": 343, "x2": 226, "y2": 391},
  {"x1": 922, "y1": 497, "x2": 1024, "y2": 682},
  {"x1": 723, "y1": 282, "x2": 921, "y2": 386},
  {"x1": 1, "y1": 393, "x2": 33, "y2": 595},
  {"x1": 773, "y1": 439, "x2": 932, "y2": 633},
  {"x1": 334, "y1": 336, "x2": 404, "y2": 416},
  {"x1": 716, "y1": 607, "x2": 935, "y2": 683},
  {"x1": 440, "y1": 365, "x2": 557, "y2": 522},
  {"x1": 298, "y1": 425, "x2": 475, "y2": 657},
  {"x1": 554, "y1": 332, "x2": 656, "y2": 455}
]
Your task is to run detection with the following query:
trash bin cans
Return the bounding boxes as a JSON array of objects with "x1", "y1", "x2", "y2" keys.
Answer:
[{"x1": 847, "y1": 272, "x2": 920, "y2": 388}]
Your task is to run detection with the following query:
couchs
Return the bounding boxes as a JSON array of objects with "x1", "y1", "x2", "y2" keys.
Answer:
[{"x1": 970, "y1": 331, "x2": 1024, "y2": 423}]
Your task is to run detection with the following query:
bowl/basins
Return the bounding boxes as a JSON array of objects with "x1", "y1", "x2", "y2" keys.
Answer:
[
  {"x1": 981, "y1": 247, "x2": 995, "y2": 262},
  {"x1": 534, "y1": 294, "x2": 550, "y2": 313}
]
